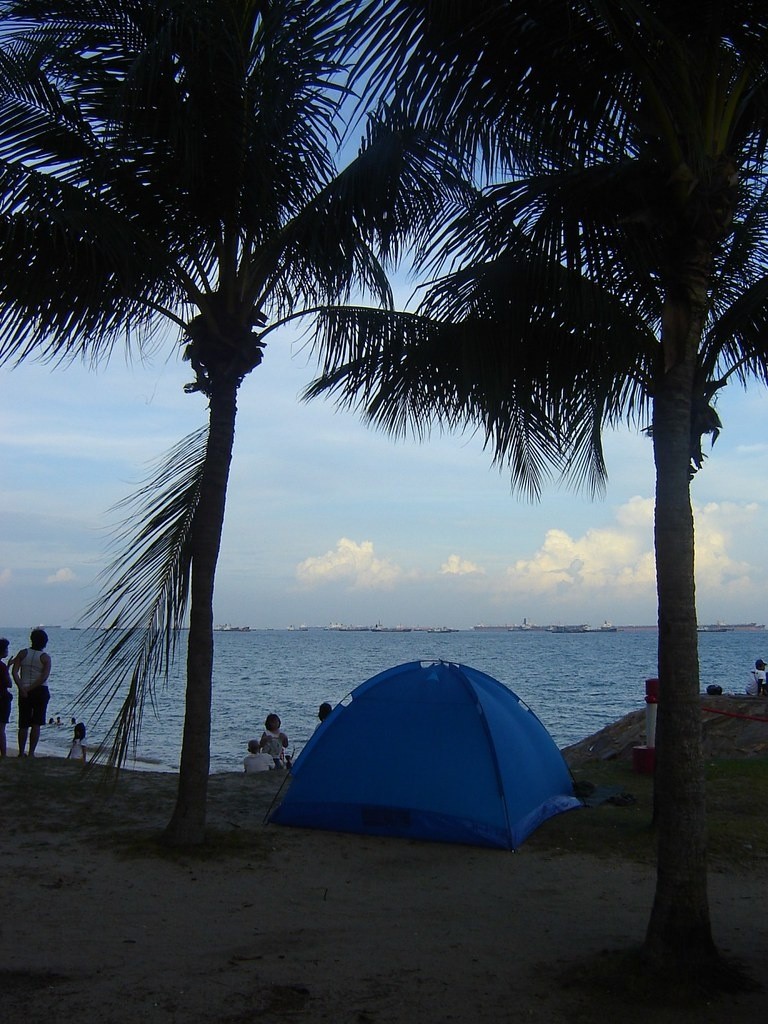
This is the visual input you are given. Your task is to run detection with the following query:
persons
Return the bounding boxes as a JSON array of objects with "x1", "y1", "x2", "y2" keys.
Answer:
[
  {"x1": 66, "y1": 723, "x2": 87, "y2": 761},
  {"x1": 243, "y1": 713, "x2": 288, "y2": 774},
  {"x1": 315, "y1": 701, "x2": 332, "y2": 730},
  {"x1": 49, "y1": 717, "x2": 77, "y2": 726},
  {"x1": 12, "y1": 629, "x2": 51, "y2": 759},
  {"x1": 746, "y1": 658, "x2": 768, "y2": 697},
  {"x1": 0, "y1": 638, "x2": 16, "y2": 758}
]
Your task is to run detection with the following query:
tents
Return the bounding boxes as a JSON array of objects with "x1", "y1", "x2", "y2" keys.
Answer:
[{"x1": 265, "y1": 662, "x2": 586, "y2": 851}]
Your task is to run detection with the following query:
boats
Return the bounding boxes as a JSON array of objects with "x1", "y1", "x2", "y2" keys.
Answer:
[
  {"x1": 584, "y1": 620, "x2": 618, "y2": 632},
  {"x1": 447, "y1": 628, "x2": 460, "y2": 633},
  {"x1": 551, "y1": 627, "x2": 583, "y2": 633},
  {"x1": 370, "y1": 623, "x2": 413, "y2": 632},
  {"x1": 546, "y1": 625, "x2": 554, "y2": 631},
  {"x1": 697, "y1": 626, "x2": 727, "y2": 632},
  {"x1": 426, "y1": 626, "x2": 451, "y2": 632},
  {"x1": 213, "y1": 623, "x2": 253, "y2": 631},
  {"x1": 508, "y1": 624, "x2": 534, "y2": 632},
  {"x1": 296, "y1": 626, "x2": 310, "y2": 631},
  {"x1": 723, "y1": 625, "x2": 735, "y2": 631},
  {"x1": 338, "y1": 622, "x2": 370, "y2": 631}
]
